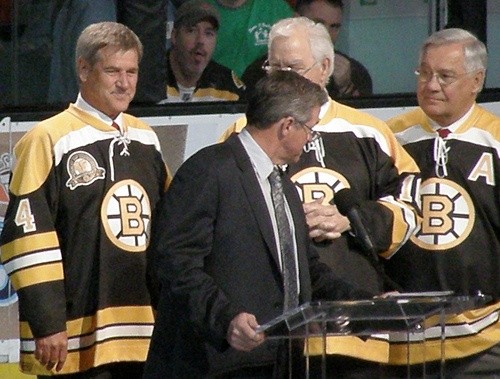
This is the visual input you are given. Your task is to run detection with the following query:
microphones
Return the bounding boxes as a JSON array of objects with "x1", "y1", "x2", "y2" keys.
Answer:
[{"x1": 333, "y1": 188, "x2": 379, "y2": 263}]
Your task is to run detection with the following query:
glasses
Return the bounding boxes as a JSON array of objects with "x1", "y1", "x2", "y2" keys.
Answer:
[
  {"x1": 414, "y1": 66, "x2": 483, "y2": 83},
  {"x1": 285, "y1": 114, "x2": 320, "y2": 142},
  {"x1": 262, "y1": 58, "x2": 320, "y2": 77}
]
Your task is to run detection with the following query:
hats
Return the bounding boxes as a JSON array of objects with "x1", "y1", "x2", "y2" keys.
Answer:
[{"x1": 174, "y1": 1, "x2": 219, "y2": 32}]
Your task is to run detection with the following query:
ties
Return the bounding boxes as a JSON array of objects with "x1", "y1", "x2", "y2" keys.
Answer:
[
  {"x1": 436, "y1": 128, "x2": 451, "y2": 138},
  {"x1": 112, "y1": 121, "x2": 120, "y2": 130},
  {"x1": 268, "y1": 167, "x2": 298, "y2": 320}
]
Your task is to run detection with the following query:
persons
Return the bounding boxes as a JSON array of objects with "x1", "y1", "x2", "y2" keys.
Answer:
[
  {"x1": 142, "y1": 71, "x2": 407, "y2": 379},
  {"x1": 0, "y1": 0, "x2": 500, "y2": 379}
]
